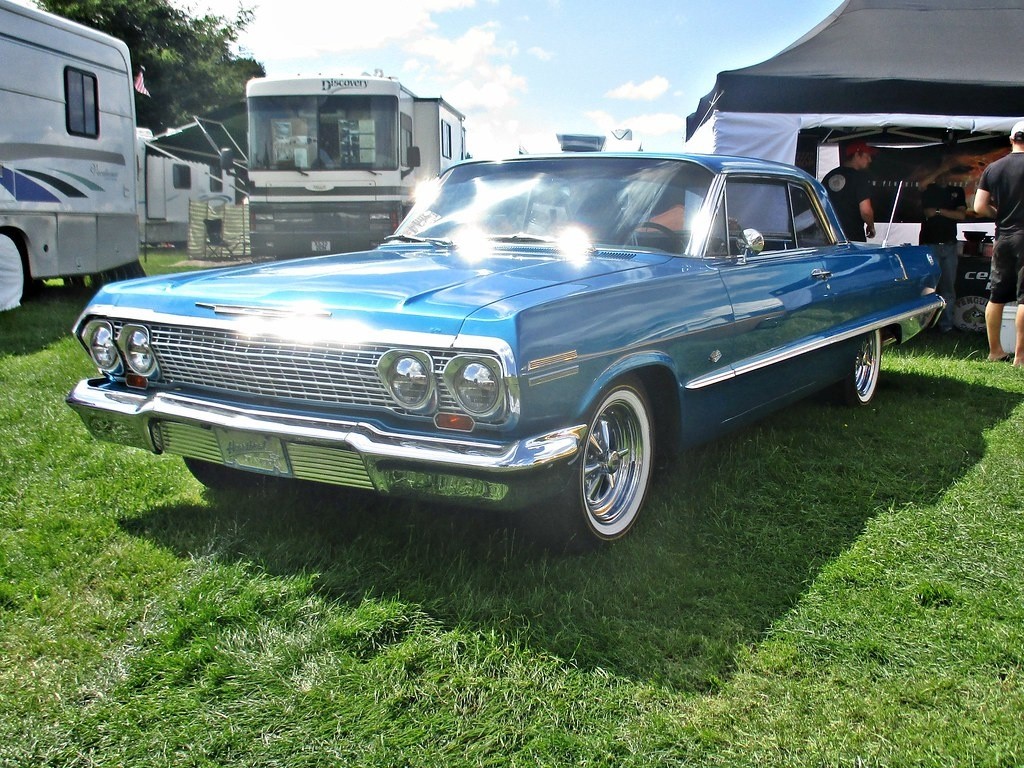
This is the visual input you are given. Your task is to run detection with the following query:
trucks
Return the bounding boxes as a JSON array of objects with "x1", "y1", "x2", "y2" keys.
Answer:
[{"x1": 218, "y1": 76, "x2": 465, "y2": 263}]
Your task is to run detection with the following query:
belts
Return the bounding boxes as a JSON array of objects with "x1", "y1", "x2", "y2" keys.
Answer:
[{"x1": 934, "y1": 239, "x2": 955, "y2": 245}]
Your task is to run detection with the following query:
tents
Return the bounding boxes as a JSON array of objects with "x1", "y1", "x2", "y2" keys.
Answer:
[{"x1": 687, "y1": 0, "x2": 1024, "y2": 246}]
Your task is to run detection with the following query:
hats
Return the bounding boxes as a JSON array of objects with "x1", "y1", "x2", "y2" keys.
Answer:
[
  {"x1": 1010, "y1": 121, "x2": 1023, "y2": 140},
  {"x1": 845, "y1": 138, "x2": 878, "y2": 155}
]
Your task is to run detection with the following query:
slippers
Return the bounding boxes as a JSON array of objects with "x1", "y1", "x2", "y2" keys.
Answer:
[{"x1": 1001, "y1": 355, "x2": 1010, "y2": 361}]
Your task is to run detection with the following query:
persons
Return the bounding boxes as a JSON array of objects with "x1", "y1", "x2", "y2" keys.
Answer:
[
  {"x1": 822, "y1": 140, "x2": 877, "y2": 242},
  {"x1": 917, "y1": 159, "x2": 967, "y2": 336},
  {"x1": 974, "y1": 121, "x2": 1024, "y2": 366}
]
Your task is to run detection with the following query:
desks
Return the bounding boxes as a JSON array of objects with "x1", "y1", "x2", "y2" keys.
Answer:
[{"x1": 953, "y1": 253, "x2": 992, "y2": 334}]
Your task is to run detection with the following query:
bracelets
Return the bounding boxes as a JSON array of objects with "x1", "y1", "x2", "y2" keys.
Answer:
[{"x1": 936, "y1": 208, "x2": 940, "y2": 216}]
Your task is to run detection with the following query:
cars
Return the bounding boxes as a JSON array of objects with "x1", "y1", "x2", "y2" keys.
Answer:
[{"x1": 67, "y1": 156, "x2": 947, "y2": 547}]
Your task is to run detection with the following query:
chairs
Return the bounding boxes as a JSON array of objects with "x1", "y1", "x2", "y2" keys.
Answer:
[{"x1": 203, "y1": 219, "x2": 239, "y2": 261}]
